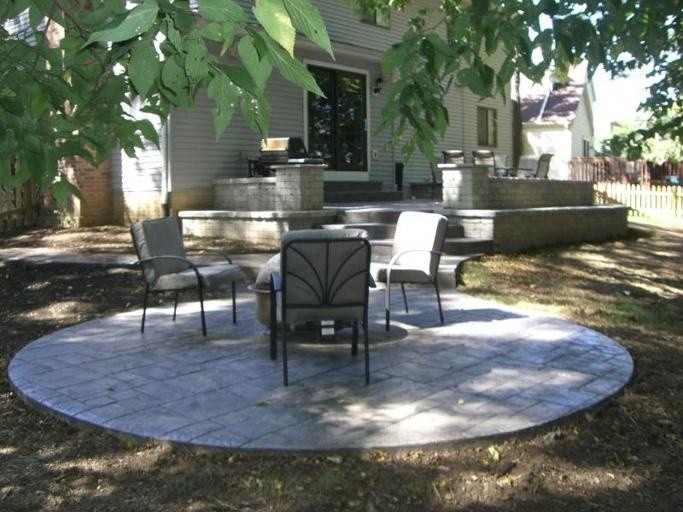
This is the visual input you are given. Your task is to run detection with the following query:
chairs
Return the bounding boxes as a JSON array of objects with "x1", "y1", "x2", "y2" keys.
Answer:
[
  {"x1": 129, "y1": 216, "x2": 245, "y2": 335},
  {"x1": 428, "y1": 149, "x2": 555, "y2": 200},
  {"x1": 270, "y1": 212, "x2": 450, "y2": 386}
]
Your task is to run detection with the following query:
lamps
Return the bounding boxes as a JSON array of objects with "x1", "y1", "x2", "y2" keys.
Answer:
[{"x1": 374, "y1": 78, "x2": 384, "y2": 93}]
